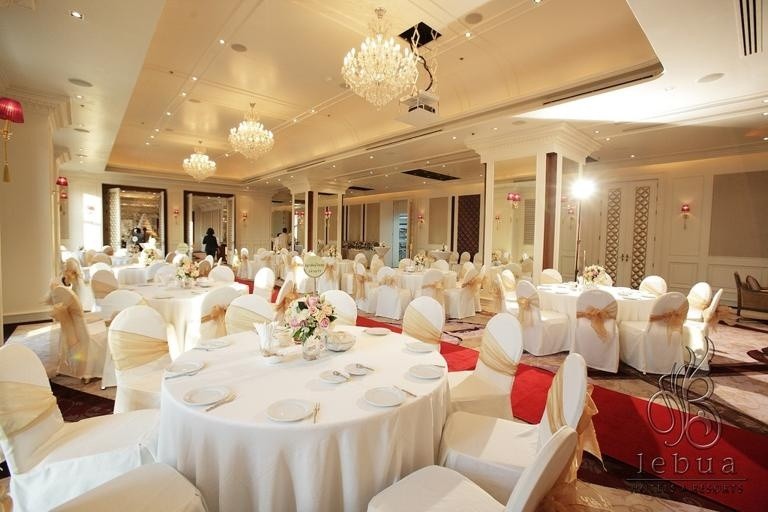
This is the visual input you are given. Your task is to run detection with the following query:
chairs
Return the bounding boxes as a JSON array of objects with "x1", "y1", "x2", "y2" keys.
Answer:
[
  {"x1": 443, "y1": 352, "x2": 587, "y2": 508},
  {"x1": 0, "y1": 343, "x2": 156, "y2": 512},
  {"x1": 274, "y1": 279, "x2": 293, "y2": 307},
  {"x1": 746, "y1": 275, "x2": 768, "y2": 298},
  {"x1": 205, "y1": 255, "x2": 213, "y2": 269},
  {"x1": 258, "y1": 251, "x2": 273, "y2": 270},
  {"x1": 375, "y1": 266, "x2": 411, "y2": 320},
  {"x1": 445, "y1": 262, "x2": 475, "y2": 317},
  {"x1": 166, "y1": 251, "x2": 176, "y2": 263},
  {"x1": 494, "y1": 273, "x2": 517, "y2": 313},
  {"x1": 430, "y1": 259, "x2": 448, "y2": 278},
  {"x1": 198, "y1": 261, "x2": 211, "y2": 277},
  {"x1": 101, "y1": 245, "x2": 114, "y2": 257},
  {"x1": 355, "y1": 253, "x2": 368, "y2": 269},
  {"x1": 415, "y1": 270, "x2": 445, "y2": 306},
  {"x1": 91, "y1": 253, "x2": 107, "y2": 266},
  {"x1": 473, "y1": 252, "x2": 481, "y2": 311},
  {"x1": 399, "y1": 258, "x2": 417, "y2": 270},
  {"x1": 686, "y1": 282, "x2": 712, "y2": 310},
  {"x1": 638, "y1": 275, "x2": 667, "y2": 296},
  {"x1": 315, "y1": 291, "x2": 357, "y2": 325},
  {"x1": 200, "y1": 286, "x2": 241, "y2": 340},
  {"x1": 50, "y1": 286, "x2": 109, "y2": 384},
  {"x1": 459, "y1": 252, "x2": 471, "y2": 264},
  {"x1": 50, "y1": 463, "x2": 205, "y2": 512},
  {"x1": 366, "y1": 427, "x2": 578, "y2": 512},
  {"x1": 540, "y1": 269, "x2": 563, "y2": 282},
  {"x1": 250, "y1": 268, "x2": 274, "y2": 304},
  {"x1": 155, "y1": 264, "x2": 175, "y2": 282},
  {"x1": 577, "y1": 268, "x2": 612, "y2": 286},
  {"x1": 370, "y1": 254, "x2": 385, "y2": 275},
  {"x1": 685, "y1": 288, "x2": 723, "y2": 368},
  {"x1": 91, "y1": 270, "x2": 146, "y2": 338},
  {"x1": 514, "y1": 281, "x2": 570, "y2": 356},
  {"x1": 217, "y1": 256, "x2": 222, "y2": 267},
  {"x1": 501, "y1": 268, "x2": 517, "y2": 292},
  {"x1": 208, "y1": 266, "x2": 234, "y2": 282},
  {"x1": 621, "y1": 293, "x2": 688, "y2": 373},
  {"x1": 83, "y1": 249, "x2": 99, "y2": 265},
  {"x1": 257, "y1": 248, "x2": 267, "y2": 254},
  {"x1": 341, "y1": 259, "x2": 353, "y2": 295},
  {"x1": 569, "y1": 290, "x2": 619, "y2": 372},
  {"x1": 450, "y1": 314, "x2": 523, "y2": 421},
  {"x1": 353, "y1": 263, "x2": 375, "y2": 314},
  {"x1": 225, "y1": 295, "x2": 275, "y2": 334},
  {"x1": 232, "y1": 249, "x2": 241, "y2": 272},
  {"x1": 119, "y1": 267, "x2": 144, "y2": 285},
  {"x1": 400, "y1": 295, "x2": 445, "y2": 351},
  {"x1": 733, "y1": 272, "x2": 768, "y2": 324},
  {"x1": 64, "y1": 258, "x2": 94, "y2": 311},
  {"x1": 448, "y1": 252, "x2": 459, "y2": 266},
  {"x1": 237, "y1": 248, "x2": 255, "y2": 281}
]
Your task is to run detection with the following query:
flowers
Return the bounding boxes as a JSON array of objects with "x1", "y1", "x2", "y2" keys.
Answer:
[
  {"x1": 583, "y1": 265, "x2": 605, "y2": 284},
  {"x1": 141, "y1": 248, "x2": 154, "y2": 266},
  {"x1": 284, "y1": 292, "x2": 335, "y2": 354},
  {"x1": 414, "y1": 253, "x2": 426, "y2": 264},
  {"x1": 177, "y1": 257, "x2": 200, "y2": 284},
  {"x1": 328, "y1": 246, "x2": 335, "y2": 254}
]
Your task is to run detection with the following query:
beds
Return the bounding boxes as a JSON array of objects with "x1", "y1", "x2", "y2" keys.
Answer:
[{"x1": 162, "y1": 324, "x2": 449, "y2": 509}]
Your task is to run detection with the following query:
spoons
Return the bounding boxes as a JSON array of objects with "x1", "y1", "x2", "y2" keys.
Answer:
[
  {"x1": 356, "y1": 364, "x2": 373, "y2": 371},
  {"x1": 333, "y1": 371, "x2": 349, "y2": 380}
]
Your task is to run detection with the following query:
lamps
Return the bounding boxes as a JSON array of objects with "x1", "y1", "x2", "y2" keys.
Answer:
[
  {"x1": 53, "y1": 176, "x2": 65, "y2": 195},
  {"x1": 60, "y1": 192, "x2": 68, "y2": 215},
  {"x1": 228, "y1": 103, "x2": 274, "y2": 161},
  {"x1": 0, "y1": 97, "x2": 24, "y2": 181},
  {"x1": 181, "y1": 144, "x2": 215, "y2": 182},
  {"x1": 340, "y1": 6, "x2": 421, "y2": 108},
  {"x1": 507, "y1": 193, "x2": 522, "y2": 209},
  {"x1": 398, "y1": 22, "x2": 441, "y2": 130},
  {"x1": 682, "y1": 204, "x2": 690, "y2": 230}
]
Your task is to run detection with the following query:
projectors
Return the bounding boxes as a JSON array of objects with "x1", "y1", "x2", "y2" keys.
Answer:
[{"x1": 394, "y1": 89, "x2": 440, "y2": 127}]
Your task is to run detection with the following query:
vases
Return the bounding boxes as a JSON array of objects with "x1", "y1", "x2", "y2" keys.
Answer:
[{"x1": 303, "y1": 335, "x2": 319, "y2": 361}]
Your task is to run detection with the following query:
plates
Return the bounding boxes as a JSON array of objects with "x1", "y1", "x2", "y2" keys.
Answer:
[
  {"x1": 407, "y1": 342, "x2": 434, "y2": 352},
  {"x1": 364, "y1": 387, "x2": 405, "y2": 407},
  {"x1": 319, "y1": 370, "x2": 348, "y2": 383},
  {"x1": 152, "y1": 294, "x2": 172, "y2": 299},
  {"x1": 165, "y1": 360, "x2": 204, "y2": 374},
  {"x1": 346, "y1": 363, "x2": 367, "y2": 375},
  {"x1": 259, "y1": 349, "x2": 287, "y2": 362},
  {"x1": 366, "y1": 327, "x2": 390, "y2": 335},
  {"x1": 204, "y1": 340, "x2": 230, "y2": 349},
  {"x1": 265, "y1": 398, "x2": 314, "y2": 421},
  {"x1": 184, "y1": 385, "x2": 230, "y2": 405},
  {"x1": 408, "y1": 363, "x2": 443, "y2": 379}
]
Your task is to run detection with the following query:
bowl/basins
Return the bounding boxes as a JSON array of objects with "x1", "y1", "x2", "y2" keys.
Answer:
[{"x1": 326, "y1": 332, "x2": 355, "y2": 352}]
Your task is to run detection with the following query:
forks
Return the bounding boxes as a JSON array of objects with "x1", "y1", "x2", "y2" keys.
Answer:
[
  {"x1": 314, "y1": 403, "x2": 321, "y2": 424},
  {"x1": 206, "y1": 394, "x2": 237, "y2": 411}
]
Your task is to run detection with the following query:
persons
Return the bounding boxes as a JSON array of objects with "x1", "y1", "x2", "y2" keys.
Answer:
[
  {"x1": 280, "y1": 228, "x2": 290, "y2": 243},
  {"x1": 203, "y1": 228, "x2": 217, "y2": 258},
  {"x1": 143, "y1": 228, "x2": 149, "y2": 242}
]
